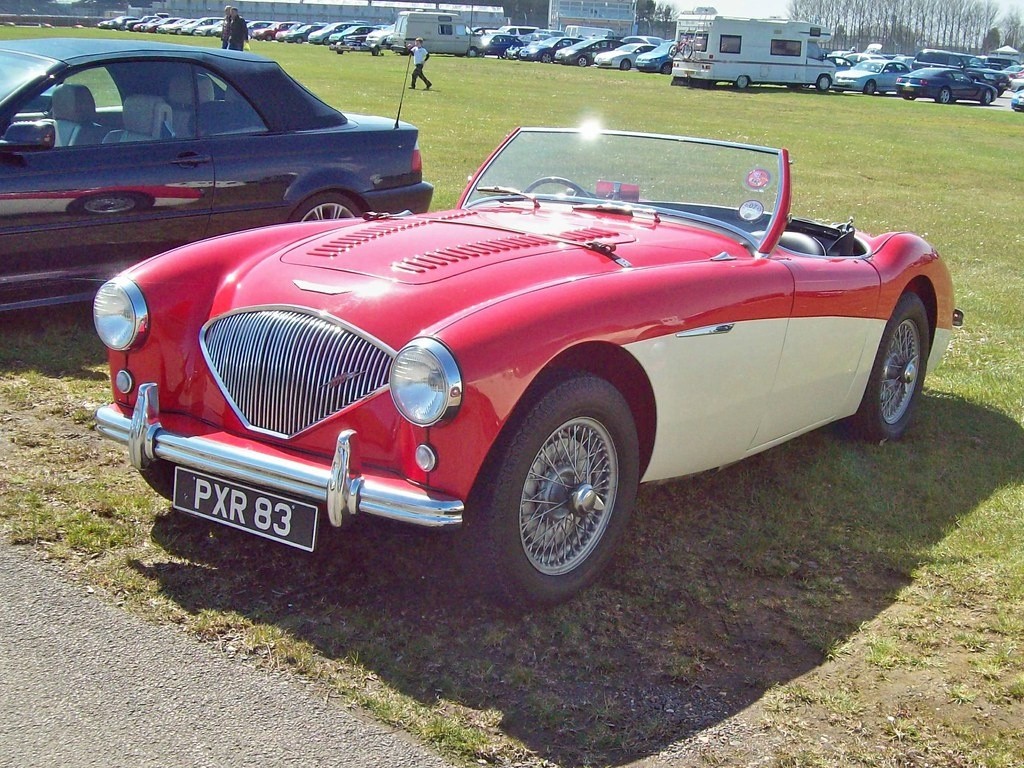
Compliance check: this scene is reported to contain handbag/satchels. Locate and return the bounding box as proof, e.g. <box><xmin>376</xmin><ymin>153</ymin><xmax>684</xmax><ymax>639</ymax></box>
<box><xmin>243</xmin><ymin>43</ymin><xmax>250</xmax><ymax>51</ymax></box>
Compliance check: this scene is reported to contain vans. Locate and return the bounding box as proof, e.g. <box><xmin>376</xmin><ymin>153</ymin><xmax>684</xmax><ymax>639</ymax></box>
<box><xmin>565</xmin><ymin>24</ymin><xmax>617</xmax><ymax>40</ymax></box>
<box><xmin>970</xmin><ymin>55</ymin><xmax>1022</xmax><ymax>71</ymax></box>
<box><xmin>392</xmin><ymin>8</ymin><xmax>484</xmax><ymax>58</ymax></box>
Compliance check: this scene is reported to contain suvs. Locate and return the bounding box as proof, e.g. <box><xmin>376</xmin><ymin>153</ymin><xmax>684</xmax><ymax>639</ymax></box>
<box><xmin>911</xmin><ymin>48</ymin><xmax>1011</xmax><ymax>98</ymax></box>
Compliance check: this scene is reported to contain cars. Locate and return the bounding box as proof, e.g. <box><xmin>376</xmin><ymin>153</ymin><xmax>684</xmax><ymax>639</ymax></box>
<box><xmin>554</xmin><ymin>37</ymin><xmax>629</xmax><ymax>67</ymax></box>
<box><xmin>825</xmin><ymin>42</ymin><xmax>916</xmax><ymax>71</ymax></box>
<box><xmin>1011</xmin><ymin>87</ymin><xmax>1024</xmax><ymax>112</ymax></box>
<box><xmin>834</xmin><ymin>59</ymin><xmax>913</xmax><ymax>96</ymax></box>
<box><xmin>594</xmin><ymin>42</ymin><xmax>658</xmax><ymax>71</ymax></box>
<box><xmin>519</xmin><ymin>29</ymin><xmax>564</xmax><ymax>48</ymax></box>
<box><xmin>97</xmin><ymin>12</ymin><xmax>397</xmax><ymax>53</ymax></box>
<box><xmin>480</xmin><ymin>25</ymin><xmax>540</xmax><ymax>60</ymax></box>
<box><xmin>0</xmin><ymin>37</ymin><xmax>434</xmax><ymax>313</ymax></box>
<box><xmin>635</xmin><ymin>41</ymin><xmax>676</xmax><ymax>76</ymax></box>
<box><xmin>519</xmin><ymin>35</ymin><xmax>587</xmax><ymax>63</ymax></box>
<box><xmin>999</xmin><ymin>64</ymin><xmax>1024</xmax><ymax>93</ymax></box>
<box><xmin>621</xmin><ymin>35</ymin><xmax>667</xmax><ymax>47</ymax></box>
<box><xmin>895</xmin><ymin>66</ymin><xmax>999</xmax><ymax>107</ymax></box>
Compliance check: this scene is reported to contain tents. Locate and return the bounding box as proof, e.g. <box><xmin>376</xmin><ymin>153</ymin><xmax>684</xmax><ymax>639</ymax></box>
<box><xmin>991</xmin><ymin>45</ymin><xmax>1019</xmax><ymax>57</ymax></box>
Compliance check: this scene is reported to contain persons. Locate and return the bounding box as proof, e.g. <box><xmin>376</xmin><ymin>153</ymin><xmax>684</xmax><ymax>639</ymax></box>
<box><xmin>221</xmin><ymin>6</ymin><xmax>249</xmax><ymax>51</ymax></box>
<box><xmin>408</xmin><ymin>37</ymin><xmax>432</xmax><ymax>90</ymax></box>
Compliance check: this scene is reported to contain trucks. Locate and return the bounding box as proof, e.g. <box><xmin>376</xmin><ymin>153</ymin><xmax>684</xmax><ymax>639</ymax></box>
<box><xmin>671</xmin><ymin>6</ymin><xmax>837</xmax><ymax>93</ymax></box>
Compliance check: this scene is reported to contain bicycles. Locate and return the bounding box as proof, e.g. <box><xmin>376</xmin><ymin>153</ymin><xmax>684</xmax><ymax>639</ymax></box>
<box><xmin>667</xmin><ymin>33</ymin><xmax>694</xmax><ymax>60</ymax></box>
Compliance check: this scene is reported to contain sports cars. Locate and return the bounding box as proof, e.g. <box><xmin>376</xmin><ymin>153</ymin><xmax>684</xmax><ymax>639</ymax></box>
<box><xmin>93</xmin><ymin>127</ymin><xmax>966</xmax><ymax>612</ymax></box>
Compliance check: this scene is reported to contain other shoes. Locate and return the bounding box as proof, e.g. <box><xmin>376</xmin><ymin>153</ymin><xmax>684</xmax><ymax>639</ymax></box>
<box><xmin>409</xmin><ymin>86</ymin><xmax>415</xmax><ymax>89</ymax></box>
<box><xmin>424</xmin><ymin>83</ymin><xmax>431</xmax><ymax>90</ymax></box>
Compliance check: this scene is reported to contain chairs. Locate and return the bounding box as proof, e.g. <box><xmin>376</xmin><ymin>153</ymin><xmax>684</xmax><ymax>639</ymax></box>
<box><xmin>750</xmin><ymin>227</ymin><xmax>827</xmax><ymax>257</ymax></box>
<box><xmin>49</xmin><ymin>73</ymin><xmax>263</xmax><ymax>146</ymax></box>
